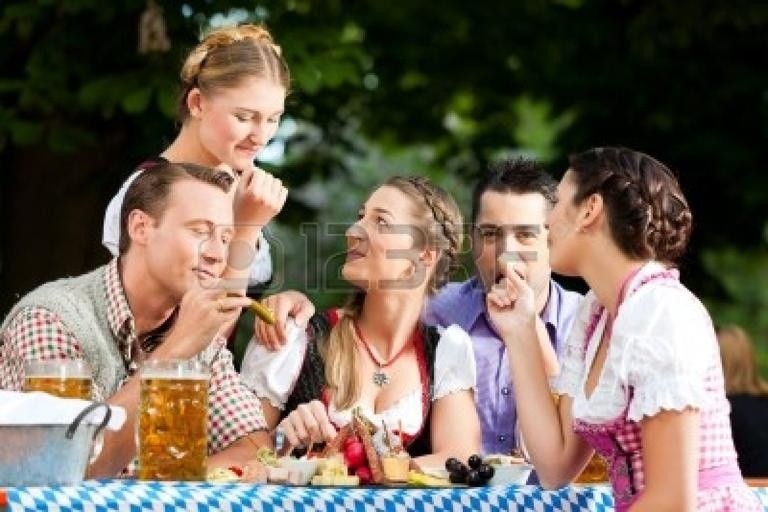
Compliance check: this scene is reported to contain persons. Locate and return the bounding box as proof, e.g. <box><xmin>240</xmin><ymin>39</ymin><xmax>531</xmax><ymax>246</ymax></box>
<box><xmin>102</xmin><ymin>21</ymin><xmax>301</xmax><ymax>374</ymax></box>
<box><xmin>712</xmin><ymin>322</ymin><xmax>768</xmax><ymax>478</ymax></box>
<box><xmin>485</xmin><ymin>146</ymin><xmax>768</xmax><ymax>512</ymax></box>
<box><xmin>0</xmin><ymin>161</ymin><xmax>275</xmax><ymax>488</ymax></box>
<box><xmin>238</xmin><ymin>172</ymin><xmax>484</xmax><ymax>475</ymax></box>
<box><xmin>251</xmin><ymin>153</ymin><xmax>587</xmax><ymax>467</ymax></box>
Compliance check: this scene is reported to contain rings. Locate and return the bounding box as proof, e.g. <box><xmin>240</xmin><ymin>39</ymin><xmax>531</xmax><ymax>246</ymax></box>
<box><xmin>216</xmin><ymin>298</ymin><xmax>227</xmax><ymax>312</ymax></box>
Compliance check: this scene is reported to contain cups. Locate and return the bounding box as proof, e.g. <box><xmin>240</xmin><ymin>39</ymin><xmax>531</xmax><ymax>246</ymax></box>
<box><xmin>136</xmin><ymin>360</ymin><xmax>212</xmax><ymax>482</ymax></box>
<box><xmin>22</xmin><ymin>357</ymin><xmax>93</xmax><ymax>401</ymax></box>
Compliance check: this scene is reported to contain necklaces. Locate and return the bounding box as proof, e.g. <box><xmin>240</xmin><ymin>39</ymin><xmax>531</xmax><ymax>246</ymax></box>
<box><xmin>600</xmin><ymin>261</ymin><xmax>649</xmax><ymax>361</ymax></box>
<box><xmin>348</xmin><ymin>308</ymin><xmax>419</xmax><ymax>391</ymax></box>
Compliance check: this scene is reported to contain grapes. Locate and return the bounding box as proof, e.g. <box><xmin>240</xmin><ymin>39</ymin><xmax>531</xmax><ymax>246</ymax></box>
<box><xmin>445</xmin><ymin>454</ymin><xmax>495</xmax><ymax>488</ymax></box>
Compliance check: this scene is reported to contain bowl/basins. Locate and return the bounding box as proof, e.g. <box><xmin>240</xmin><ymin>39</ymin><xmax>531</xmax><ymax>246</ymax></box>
<box><xmin>1</xmin><ymin>402</ymin><xmax>113</xmax><ymax>485</ymax></box>
<box><xmin>279</xmin><ymin>460</ymin><xmax>317</xmax><ymax>485</ymax></box>
<box><xmin>487</xmin><ymin>464</ymin><xmax>536</xmax><ymax>487</ymax></box>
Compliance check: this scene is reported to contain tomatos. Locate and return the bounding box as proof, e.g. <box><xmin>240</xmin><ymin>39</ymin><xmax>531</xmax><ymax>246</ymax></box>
<box><xmin>342</xmin><ymin>435</ymin><xmax>371</xmax><ymax>483</ymax></box>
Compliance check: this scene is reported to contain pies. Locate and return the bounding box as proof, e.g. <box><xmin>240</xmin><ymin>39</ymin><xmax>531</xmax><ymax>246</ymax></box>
<box><xmin>225</xmin><ymin>293</ymin><xmax>275</xmax><ymax>325</ymax></box>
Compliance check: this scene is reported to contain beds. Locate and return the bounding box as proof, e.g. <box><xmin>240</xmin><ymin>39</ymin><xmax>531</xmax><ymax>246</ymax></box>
<box><xmin>0</xmin><ymin>465</ymin><xmax>618</xmax><ymax>512</ymax></box>
